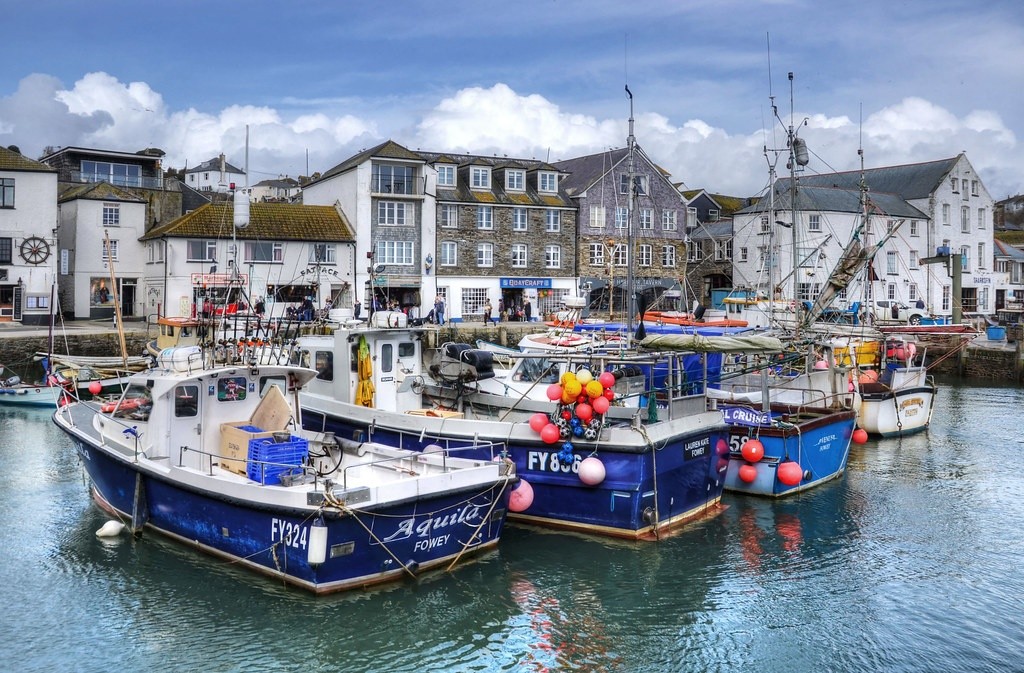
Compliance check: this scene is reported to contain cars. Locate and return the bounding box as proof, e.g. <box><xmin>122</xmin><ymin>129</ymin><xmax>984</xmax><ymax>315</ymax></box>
<box><xmin>862</xmin><ymin>300</ymin><xmax>930</xmax><ymax>324</ymax></box>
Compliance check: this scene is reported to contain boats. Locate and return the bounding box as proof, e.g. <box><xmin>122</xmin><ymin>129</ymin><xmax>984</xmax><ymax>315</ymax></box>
<box><xmin>0</xmin><ymin>231</ymin><xmax>158</xmax><ymax>408</ymax></box>
<box><xmin>49</xmin><ymin>65</ymin><xmax>968</xmax><ymax>594</ymax></box>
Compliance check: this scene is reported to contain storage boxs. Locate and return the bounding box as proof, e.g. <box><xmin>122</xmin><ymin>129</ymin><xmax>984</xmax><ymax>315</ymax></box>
<box><xmin>409</xmin><ymin>409</ymin><xmax>465</xmax><ymax>419</ymax></box>
<box><xmin>987</xmin><ymin>327</ymin><xmax>1005</xmax><ymax>340</ymax></box>
<box><xmin>219</xmin><ymin>383</ymin><xmax>309</xmax><ymax>486</ymax></box>
<box><xmin>615</xmin><ymin>375</ymin><xmax>645</xmax><ymax>394</ymax></box>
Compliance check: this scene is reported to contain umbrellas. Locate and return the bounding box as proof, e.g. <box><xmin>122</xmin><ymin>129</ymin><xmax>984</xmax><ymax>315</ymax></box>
<box><xmin>355</xmin><ymin>336</ymin><xmax>376</xmax><ymax>406</ymax></box>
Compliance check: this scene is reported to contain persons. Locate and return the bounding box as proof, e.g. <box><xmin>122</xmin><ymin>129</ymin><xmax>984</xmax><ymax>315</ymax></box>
<box><xmin>525</xmin><ymin>300</ymin><xmax>531</xmax><ymax>322</ymax></box>
<box><xmin>498</xmin><ymin>299</ymin><xmax>504</xmax><ymax>322</ymax></box>
<box><xmin>354</xmin><ymin>301</ymin><xmax>361</xmax><ymax>319</ymax></box>
<box><xmin>287</xmin><ymin>296</ymin><xmax>332</xmax><ymax>321</ymax></box>
<box><xmin>484</xmin><ymin>298</ymin><xmax>496</xmax><ymax>326</ymax></box>
<box><xmin>916</xmin><ymin>297</ymin><xmax>924</xmax><ymax>309</ymax></box>
<box><xmin>236</xmin><ymin>297</ymin><xmax>265</xmax><ymax>315</ymax></box>
<box><xmin>433</xmin><ymin>293</ymin><xmax>445</xmax><ymax>326</ymax></box>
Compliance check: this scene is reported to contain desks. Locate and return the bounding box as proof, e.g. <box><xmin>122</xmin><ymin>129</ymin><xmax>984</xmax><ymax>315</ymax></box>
<box><xmin>824</xmin><ymin>310</ymin><xmax>846</xmax><ymax>322</ymax></box>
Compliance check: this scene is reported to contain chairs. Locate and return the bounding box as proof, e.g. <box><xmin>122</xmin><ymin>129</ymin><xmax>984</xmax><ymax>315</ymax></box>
<box><xmin>802</xmin><ymin>302</ymin><xmax>862</xmax><ymax>324</ymax></box>
